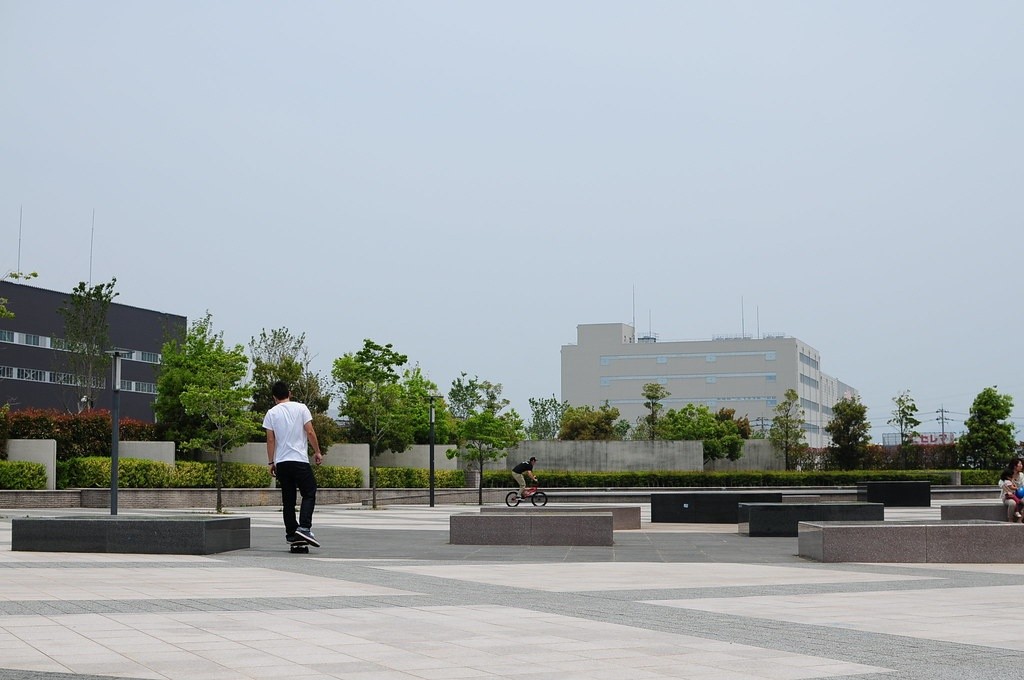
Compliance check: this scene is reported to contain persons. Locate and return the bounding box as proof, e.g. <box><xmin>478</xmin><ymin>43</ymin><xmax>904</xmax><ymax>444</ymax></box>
<box><xmin>512</xmin><ymin>457</ymin><xmax>538</xmax><ymax>501</ymax></box>
<box><xmin>997</xmin><ymin>457</ymin><xmax>1024</xmax><ymax>523</ymax></box>
<box><xmin>262</xmin><ymin>382</ymin><xmax>323</xmax><ymax>547</ymax></box>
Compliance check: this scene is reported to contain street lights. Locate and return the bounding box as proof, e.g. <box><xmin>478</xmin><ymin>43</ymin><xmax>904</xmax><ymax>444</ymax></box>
<box><xmin>104</xmin><ymin>347</ymin><xmax>135</xmax><ymax>516</ymax></box>
<box><xmin>422</xmin><ymin>395</ymin><xmax>444</xmax><ymax>508</ymax></box>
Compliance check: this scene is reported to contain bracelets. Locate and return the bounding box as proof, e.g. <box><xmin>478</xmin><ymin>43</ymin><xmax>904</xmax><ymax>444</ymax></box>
<box><xmin>268</xmin><ymin>462</ymin><xmax>273</xmax><ymax>465</ymax></box>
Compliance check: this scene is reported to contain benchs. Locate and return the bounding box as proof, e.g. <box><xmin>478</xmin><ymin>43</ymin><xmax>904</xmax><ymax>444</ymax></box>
<box><xmin>941</xmin><ymin>504</ymin><xmax>1024</xmax><ymax>523</ymax></box>
<box><xmin>12</xmin><ymin>517</ymin><xmax>251</xmax><ymax>555</ymax></box>
<box><xmin>737</xmin><ymin>502</ymin><xmax>884</xmax><ymax>537</ymax></box>
<box><xmin>651</xmin><ymin>492</ymin><xmax>783</xmax><ymax>525</ymax></box>
<box><xmin>480</xmin><ymin>506</ymin><xmax>641</xmax><ymax>530</ymax></box>
<box><xmin>450</xmin><ymin>511</ymin><xmax>614</xmax><ymax>546</ymax></box>
<box><xmin>798</xmin><ymin>520</ymin><xmax>1024</xmax><ymax>563</ymax></box>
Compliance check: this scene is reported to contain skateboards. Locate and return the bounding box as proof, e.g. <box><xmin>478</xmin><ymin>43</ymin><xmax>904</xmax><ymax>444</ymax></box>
<box><xmin>286</xmin><ymin>541</ymin><xmax>309</xmax><ymax>554</ymax></box>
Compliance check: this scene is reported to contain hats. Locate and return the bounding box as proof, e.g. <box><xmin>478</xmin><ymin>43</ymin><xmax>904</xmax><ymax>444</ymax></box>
<box><xmin>530</xmin><ymin>457</ymin><xmax>538</xmax><ymax>461</ymax></box>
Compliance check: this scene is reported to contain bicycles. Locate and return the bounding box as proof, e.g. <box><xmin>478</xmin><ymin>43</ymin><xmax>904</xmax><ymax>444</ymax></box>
<box><xmin>505</xmin><ymin>475</ymin><xmax>548</xmax><ymax>507</ymax></box>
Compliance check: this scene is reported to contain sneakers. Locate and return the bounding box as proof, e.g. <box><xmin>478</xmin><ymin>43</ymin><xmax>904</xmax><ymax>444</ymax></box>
<box><xmin>295</xmin><ymin>529</ymin><xmax>320</xmax><ymax>548</ymax></box>
<box><xmin>285</xmin><ymin>536</ymin><xmax>307</xmax><ymax>544</ymax></box>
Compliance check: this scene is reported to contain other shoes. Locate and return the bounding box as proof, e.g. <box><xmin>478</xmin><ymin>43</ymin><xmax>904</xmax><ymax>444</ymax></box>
<box><xmin>1015</xmin><ymin>511</ymin><xmax>1022</xmax><ymax>517</ymax></box>
<box><xmin>516</xmin><ymin>497</ymin><xmax>526</xmax><ymax>501</ymax></box>
